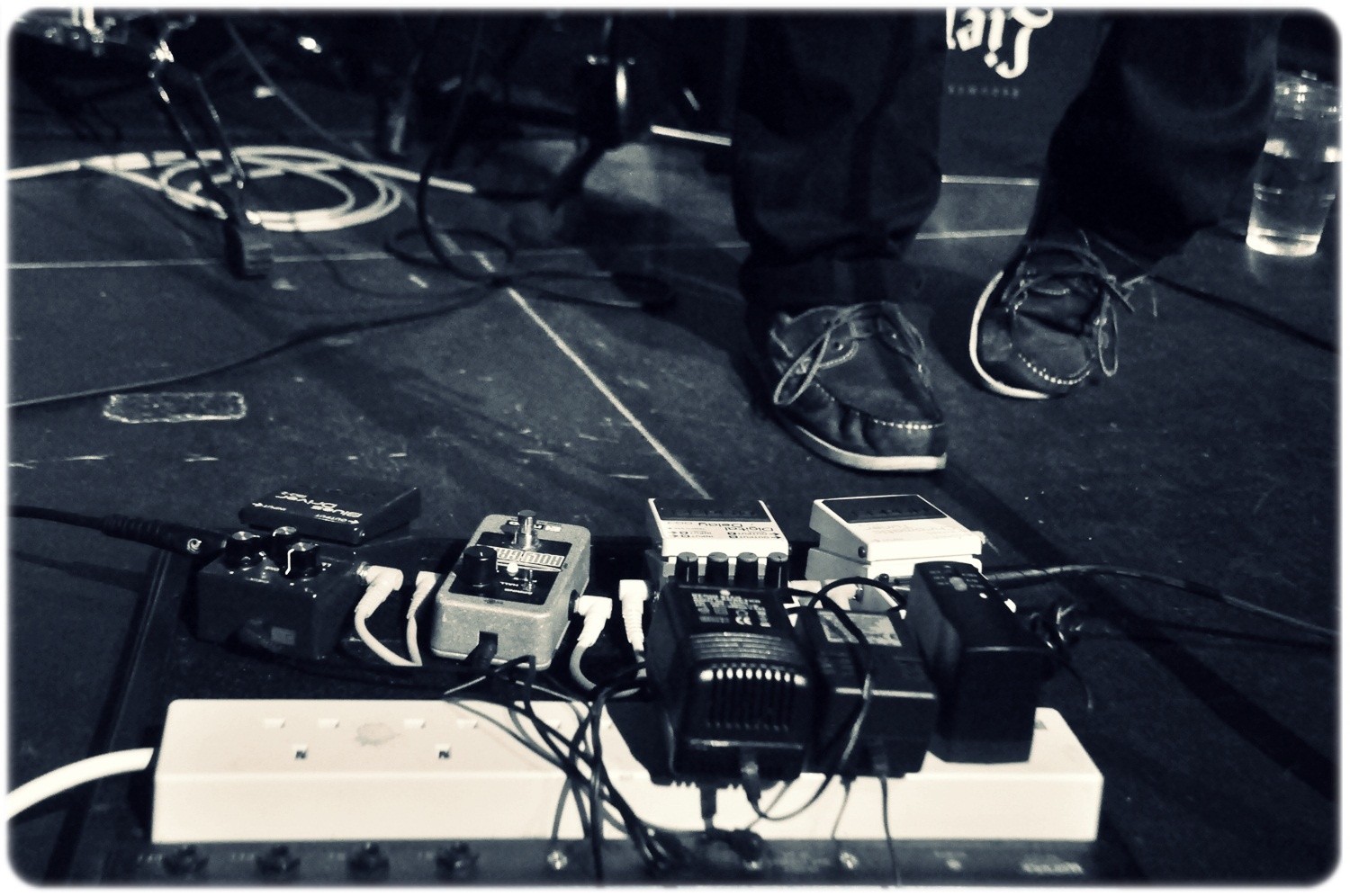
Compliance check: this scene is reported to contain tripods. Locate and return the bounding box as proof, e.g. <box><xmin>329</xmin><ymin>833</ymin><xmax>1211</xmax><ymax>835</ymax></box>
<box><xmin>462</xmin><ymin>10</ymin><xmax>737</xmax><ymax>244</ymax></box>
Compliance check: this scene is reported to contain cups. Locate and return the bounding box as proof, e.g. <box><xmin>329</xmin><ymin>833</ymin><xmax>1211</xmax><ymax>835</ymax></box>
<box><xmin>1243</xmin><ymin>69</ymin><xmax>1341</xmax><ymax>258</ymax></box>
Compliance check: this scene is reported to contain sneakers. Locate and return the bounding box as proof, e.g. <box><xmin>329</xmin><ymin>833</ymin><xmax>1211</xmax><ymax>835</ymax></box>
<box><xmin>754</xmin><ymin>300</ymin><xmax>948</xmax><ymax>473</ymax></box>
<box><xmin>968</xmin><ymin>151</ymin><xmax>1159</xmax><ymax>401</ymax></box>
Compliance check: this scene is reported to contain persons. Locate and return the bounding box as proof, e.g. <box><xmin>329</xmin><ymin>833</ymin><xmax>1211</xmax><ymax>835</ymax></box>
<box><xmin>718</xmin><ymin>7</ymin><xmax>1285</xmax><ymax>472</ymax></box>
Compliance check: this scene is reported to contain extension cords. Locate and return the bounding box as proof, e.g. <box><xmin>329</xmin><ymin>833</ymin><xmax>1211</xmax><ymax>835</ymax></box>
<box><xmin>154</xmin><ymin>698</ymin><xmax>1103</xmax><ymax>844</ymax></box>
